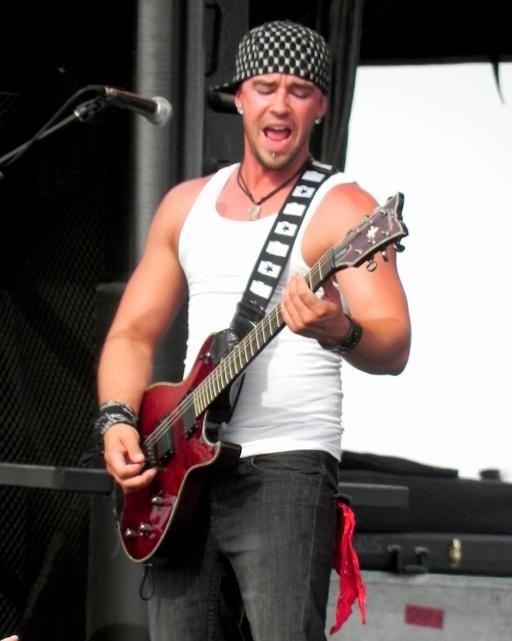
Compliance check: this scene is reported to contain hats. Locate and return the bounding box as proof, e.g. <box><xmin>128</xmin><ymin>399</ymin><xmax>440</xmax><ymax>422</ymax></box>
<box><xmin>207</xmin><ymin>20</ymin><xmax>333</xmax><ymax>95</ymax></box>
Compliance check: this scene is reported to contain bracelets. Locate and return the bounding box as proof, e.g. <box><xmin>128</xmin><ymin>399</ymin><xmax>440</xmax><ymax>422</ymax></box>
<box><xmin>95</xmin><ymin>400</ymin><xmax>139</xmax><ymax>436</ymax></box>
<box><xmin>318</xmin><ymin>314</ymin><xmax>362</xmax><ymax>355</ymax></box>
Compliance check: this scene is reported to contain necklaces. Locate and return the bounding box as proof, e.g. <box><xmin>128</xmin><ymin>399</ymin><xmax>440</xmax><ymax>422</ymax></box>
<box><xmin>236</xmin><ymin>153</ymin><xmax>311</xmax><ymax>222</ymax></box>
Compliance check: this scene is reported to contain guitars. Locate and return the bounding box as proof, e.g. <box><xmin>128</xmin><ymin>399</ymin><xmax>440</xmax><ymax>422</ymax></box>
<box><xmin>112</xmin><ymin>193</ymin><xmax>407</xmax><ymax>562</ymax></box>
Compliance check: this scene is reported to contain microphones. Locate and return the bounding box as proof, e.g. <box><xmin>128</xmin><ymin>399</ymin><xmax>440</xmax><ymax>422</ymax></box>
<box><xmin>90</xmin><ymin>85</ymin><xmax>173</xmax><ymax>128</ymax></box>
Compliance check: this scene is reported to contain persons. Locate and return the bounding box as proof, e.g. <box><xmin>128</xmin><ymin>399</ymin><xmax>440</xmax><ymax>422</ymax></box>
<box><xmin>96</xmin><ymin>21</ymin><xmax>410</xmax><ymax>641</ymax></box>
